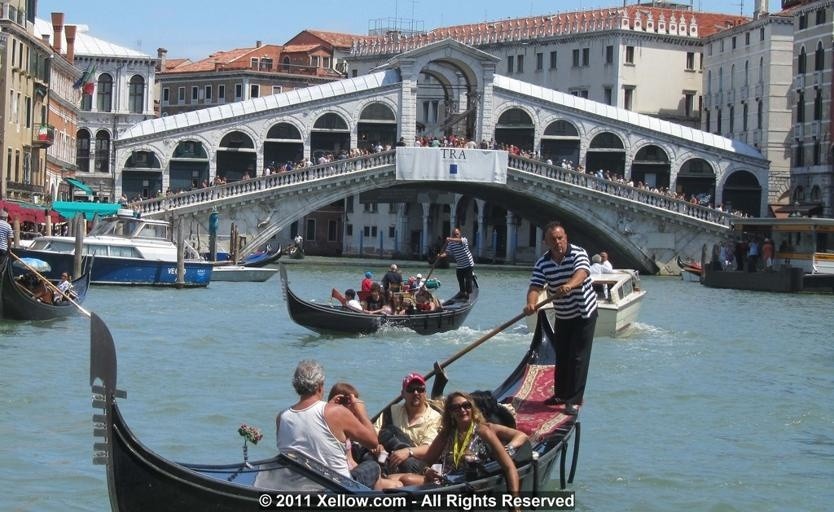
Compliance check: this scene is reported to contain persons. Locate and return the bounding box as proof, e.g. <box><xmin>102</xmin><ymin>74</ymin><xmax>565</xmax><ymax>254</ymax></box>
<box><xmin>275</xmin><ymin>357</ymin><xmax>530</xmax><ymax>512</ymax></box>
<box><xmin>0</xmin><ymin>130</ymin><xmax>794</xmax><ymax>318</ymax></box>
<box><xmin>524</xmin><ymin>222</ymin><xmax>597</xmax><ymax>416</ymax></box>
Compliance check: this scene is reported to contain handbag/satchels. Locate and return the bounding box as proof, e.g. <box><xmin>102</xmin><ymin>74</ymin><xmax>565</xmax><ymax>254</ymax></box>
<box><xmin>378</xmin><ymin>425</ymin><xmax>416</xmax><ymax>453</ymax></box>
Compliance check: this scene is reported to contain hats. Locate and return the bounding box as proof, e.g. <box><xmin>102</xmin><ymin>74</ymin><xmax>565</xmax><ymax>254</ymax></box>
<box><xmin>403</xmin><ymin>372</ymin><xmax>426</xmax><ymax>389</ymax></box>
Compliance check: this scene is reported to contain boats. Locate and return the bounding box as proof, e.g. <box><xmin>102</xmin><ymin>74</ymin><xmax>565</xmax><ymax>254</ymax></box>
<box><xmin>10</xmin><ymin>217</ymin><xmax>214</xmax><ymax>289</ymax></box>
<box><xmin>89</xmin><ymin>310</ymin><xmax>584</xmax><ymax>511</ymax></box>
<box><xmin>198</xmin><ymin>241</ymin><xmax>282</xmax><ymax>282</ymax></box>
<box><xmin>525</xmin><ymin>268</ymin><xmax>648</xmax><ymax>339</ymax></box>
<box><xmin>676</xmin><ymin>217</ymin><xmax>834</xmax><ymax>291</ymax></box>
<box><xmin>701</xmin><ymin>264</ymin><xmax>804</xmax><ymax>294</ymax></box>
<box><xmin>288</xmin><ymin>242</ymin><xmax>307</xmax><ymax>259</ymax></box>
<box><xmin>279</xmin><ymin>264</ymin><xmax>481</xmax><ymax>336</ymax></box>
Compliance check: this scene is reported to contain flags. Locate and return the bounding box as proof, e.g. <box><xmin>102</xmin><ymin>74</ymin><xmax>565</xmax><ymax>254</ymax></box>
<box><xmin>69</xmin><ymin>61</ymin><xmax>98</xmax><ymax>97</ymax></box>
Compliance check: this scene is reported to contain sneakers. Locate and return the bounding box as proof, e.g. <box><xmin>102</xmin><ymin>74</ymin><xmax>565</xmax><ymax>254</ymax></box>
<box><xmin>564</xmin><ymin>404</ymin><xmax>580</xmax><ymax>415</ymax></box>
<box><xmin>544</xmin><ymin>395</ymin><xmax>566</xmax><ymax>405</ymax></box>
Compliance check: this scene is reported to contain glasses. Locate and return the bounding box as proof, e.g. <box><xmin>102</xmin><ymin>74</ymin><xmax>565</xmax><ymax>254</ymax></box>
<box><xmin>446</xmin><ymin>401</ymin><xmax>473</xmax><ymax>413</ymax></box>
<box><xmin>406</xmin><ymin>386</ymin><xmax>426</xmax><ymax>393</ymax></box>
